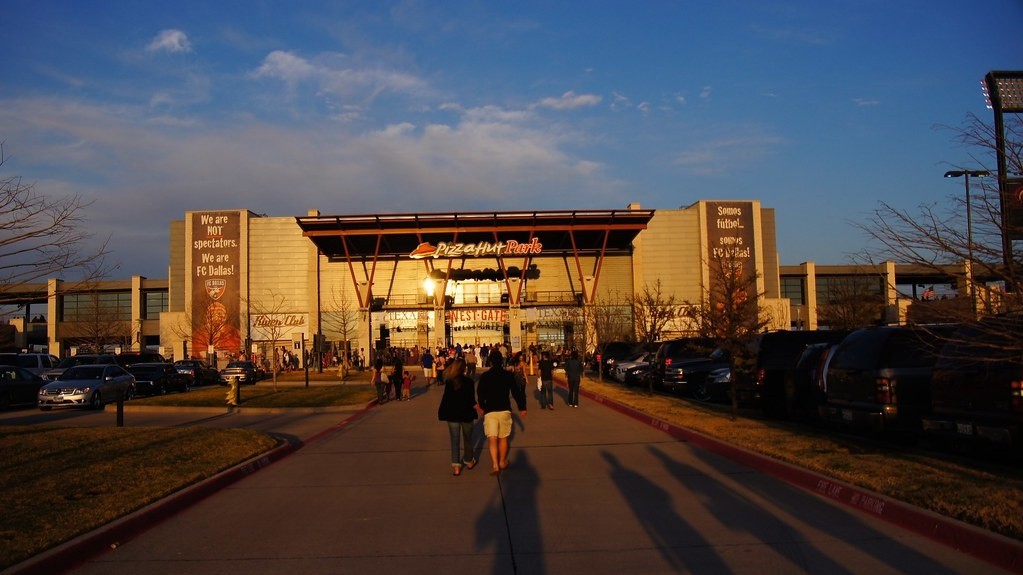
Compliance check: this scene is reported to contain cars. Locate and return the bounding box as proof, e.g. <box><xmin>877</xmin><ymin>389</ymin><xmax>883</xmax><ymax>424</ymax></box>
<box><xmin>591</xmin><ymin>323</ymin><xmax>1022</xmax><ymax>462</ymax></box>
<box><xmin>1</xmin><ymin>359</ymin><xmax>266</xmax><ymax>419</ymax></box>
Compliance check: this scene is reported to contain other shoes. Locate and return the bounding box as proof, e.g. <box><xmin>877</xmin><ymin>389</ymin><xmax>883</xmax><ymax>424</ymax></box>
<box><xmin>407</xmin><ymin>399</ymin><xmax>411</xmax><ymax>401</ymax></box>
<box><xmin>568</xmin><ymin>403</ymin><xmax>577</xmax><ymax>407</ymax></box>
<box><xmin>396</xmin><ymin>397</ymin><xmax>401</xmax><ymax>401</ymax></box>
<box><xmin>402</xmin><ymin>398</ymin><xmax>406</xmax><ymax>400</ymax></box>
<box><xmin>543</xmin><ymin>403</ymin><xmax>554</xmax><ymax>410</ymax></box>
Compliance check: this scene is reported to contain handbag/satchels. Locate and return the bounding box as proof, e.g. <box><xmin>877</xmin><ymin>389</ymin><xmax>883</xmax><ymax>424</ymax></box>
<box><xmin>437</xmin><ymin>361</ymin><xmax>442</xmax><ymax>367</ymax></box>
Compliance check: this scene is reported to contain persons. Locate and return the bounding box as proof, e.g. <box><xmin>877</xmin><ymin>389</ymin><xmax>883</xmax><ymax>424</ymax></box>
<box><xmin>167</xmin><ymin>332</ymin><xmax>597</xmax><ymax>413</ymax></box>
<box><xmin>437</xmin><ymin>358</ymin><xmax>483</xmax><ymax>475</ymax></box>
<box><xmin>476</xmin><ymin>350</ymin><xmax>527</xmax><ymax>475</ymax></box>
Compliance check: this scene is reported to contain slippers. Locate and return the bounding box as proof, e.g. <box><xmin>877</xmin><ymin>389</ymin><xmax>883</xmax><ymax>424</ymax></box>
<box><xmin>490</xmin><ymin>460</ymin><xmax>509</xmax><ymax>475</ymax></box>
<box><xmin>454</xmin><ymin>459</ymin><xmax>477</xmax><ymax>476</ymax></box>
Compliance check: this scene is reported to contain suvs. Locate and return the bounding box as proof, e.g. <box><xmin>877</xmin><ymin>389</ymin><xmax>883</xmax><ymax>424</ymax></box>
<box><xmin>39</xmin><ymin>352</ymin><xmax>168</xmax><ymax>381</ymax></box>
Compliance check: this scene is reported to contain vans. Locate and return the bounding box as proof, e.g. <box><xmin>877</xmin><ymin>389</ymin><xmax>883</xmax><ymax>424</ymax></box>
<box><xmin>2</xmin><ymin>352</ymin><xmax>60</xmax><ymax>376</ymax></box>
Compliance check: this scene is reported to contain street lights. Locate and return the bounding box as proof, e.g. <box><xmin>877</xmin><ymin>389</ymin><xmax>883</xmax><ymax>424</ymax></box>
<box><xmin>943</xmin><ymin>167</ymin><xmax>991</xmax><ymax>320</ymax></box>
